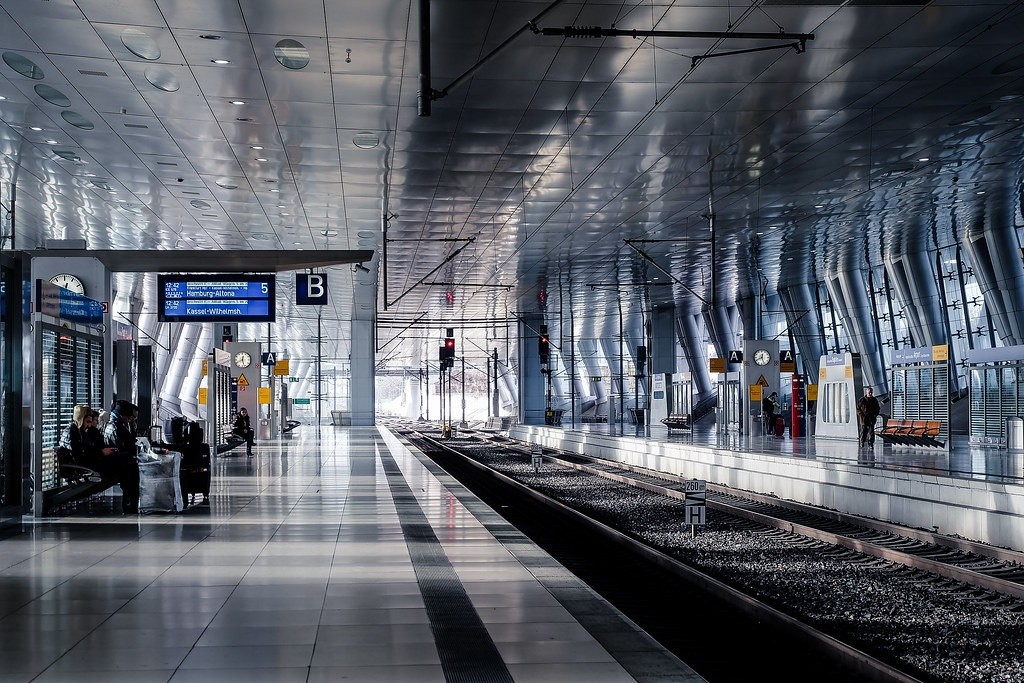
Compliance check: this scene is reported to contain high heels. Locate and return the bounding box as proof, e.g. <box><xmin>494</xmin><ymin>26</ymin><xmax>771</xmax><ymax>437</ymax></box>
<box><xmin>247</xmin><ymin>452</ymin><xmax>254</xmax><ymax>457</ymax></box>
<box><xmin>251</xmin><ymin>440</ymin><xmax>257</xmax><ymax>446</ymax></box>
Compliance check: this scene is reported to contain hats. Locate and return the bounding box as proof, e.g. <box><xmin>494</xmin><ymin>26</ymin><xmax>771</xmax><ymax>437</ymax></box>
<box><xmin>771</xmin><ymin>392</ymin><xmax>778</xmax><ymax>397</ymax></box>
<box><xmin>72</xmin><ymin>405</ymin><xmax>93</xmax><ymax>429</ymax></box>
<box><xmin>116</xmin><ymin>400</ymin><xmax>137</xmax><ymax>418</ymax></box>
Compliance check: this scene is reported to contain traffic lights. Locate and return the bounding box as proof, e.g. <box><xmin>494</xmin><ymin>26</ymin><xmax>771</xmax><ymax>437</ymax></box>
<box><xmin>538</xmin><ymin>334</ymin><xmax>549</xmax><ymax>354</ymax></box>
<box><xmin>445</xmin><ymin>337</ymin><xmax>455</xmax><ymax>358</ymax></box>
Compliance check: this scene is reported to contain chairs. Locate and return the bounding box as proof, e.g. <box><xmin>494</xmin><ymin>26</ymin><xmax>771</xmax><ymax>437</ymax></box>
<box><xmin>660</xmin><ymin>413</ymin><xmax>691</xmax><ymax>430</ymax></box>
<box><xmin>283</xmin><ymin>416</ymin><xmax>301</xmax><ymax>433</ymax></box>
<box><xmin>217</xmin><ymin>423</ymin><xmax>246</xmax><ymax>455</ymax></box>
<box><xmin>874</xmin><ymin>419</ymin><xmax>945</xmax><ymax>448</ymax></box>
<box><xmin>45</xmin><ymin>446</ymin><xmax>117</xmax><ymax>512</ymax></box>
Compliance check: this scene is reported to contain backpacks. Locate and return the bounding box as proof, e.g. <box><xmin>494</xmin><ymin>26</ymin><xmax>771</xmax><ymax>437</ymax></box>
<box><xmin>763</xmin><ymin>397</ymin><xmax>767</xmax><ymax>406</ymax></box>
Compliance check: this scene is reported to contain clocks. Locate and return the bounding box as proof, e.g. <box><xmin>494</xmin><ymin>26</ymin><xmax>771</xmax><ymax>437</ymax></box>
<box><xmin>234</xmin><ymin>351</ymin><xmax>251</xmax><ymax>369</ymax></box>
<box><xmin>49</xmin><ymin>273</ymin><xmax>85</xmax><ymax>296</ymax></box>
<box><xmin>753</xmin><ymin>349</ymin><xmax>771</xmax><ymax>366</ymax></box>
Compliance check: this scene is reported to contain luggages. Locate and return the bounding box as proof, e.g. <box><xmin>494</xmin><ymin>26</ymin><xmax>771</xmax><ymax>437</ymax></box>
<box><xmin>135</xmin><ymin>425</ymin><xmax>184</xmax><ymax>514</ymax></box>
<box><xmin>181</xmin><ymin>443</ymin><xmax>211</xmax><ymax>497</ymax></box>
<box><xmin>773</xmin><ymin>407</ymin><xmax>785</xmax><ymax>436</ymax></box>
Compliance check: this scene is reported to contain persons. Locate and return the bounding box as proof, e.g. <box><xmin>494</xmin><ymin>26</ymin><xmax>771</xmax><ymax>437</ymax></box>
<box><xmin>856</xmin><ymin>388</ymin><xmax>880</xmax><ymax>449</ymax></box>
<box><xmin>58</xmin><ymin>400</ymin><xmax>141</xmax><ymax>511</ymax></box>
<box><xmin>764</xmin><ymin>392</ymin><xmax>781</xmax><ymax>437</ymax></box>
<box><xmin>231</xmin><ymin>407</ymin><xmax>257</xmax><ymax>457</ymax></box>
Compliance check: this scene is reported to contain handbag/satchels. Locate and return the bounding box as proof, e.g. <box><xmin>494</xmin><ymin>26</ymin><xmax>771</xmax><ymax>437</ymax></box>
<box><xmin>225</xmin><ymin>435</ymin><xmax>243</xmax><ymax>444</ymax></box>
<box><xmin>183</xmin><ymin>421</ymin><xmax>204</xmax><ymax>442</ymax></box>
<box><xmin>171</xmin><ymin>416</ymin><xmax>188</xmax><ymax>442</ymax></box>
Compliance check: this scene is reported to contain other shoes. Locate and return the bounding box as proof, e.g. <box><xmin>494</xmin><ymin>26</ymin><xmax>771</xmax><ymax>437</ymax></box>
<box><xmin>767</xmin><ymin>433</ymin><xmax>773</xmax><ymax>436</ymax></box>
<box><xmin>869</xmin><ymin>443</ymin><xmax>874</xmax><ymax>449</ymax></box>
<box><xmin>860</xmin><ymin>441</ymin><xmax>864</xmax><ymax>447</ymax></box>
